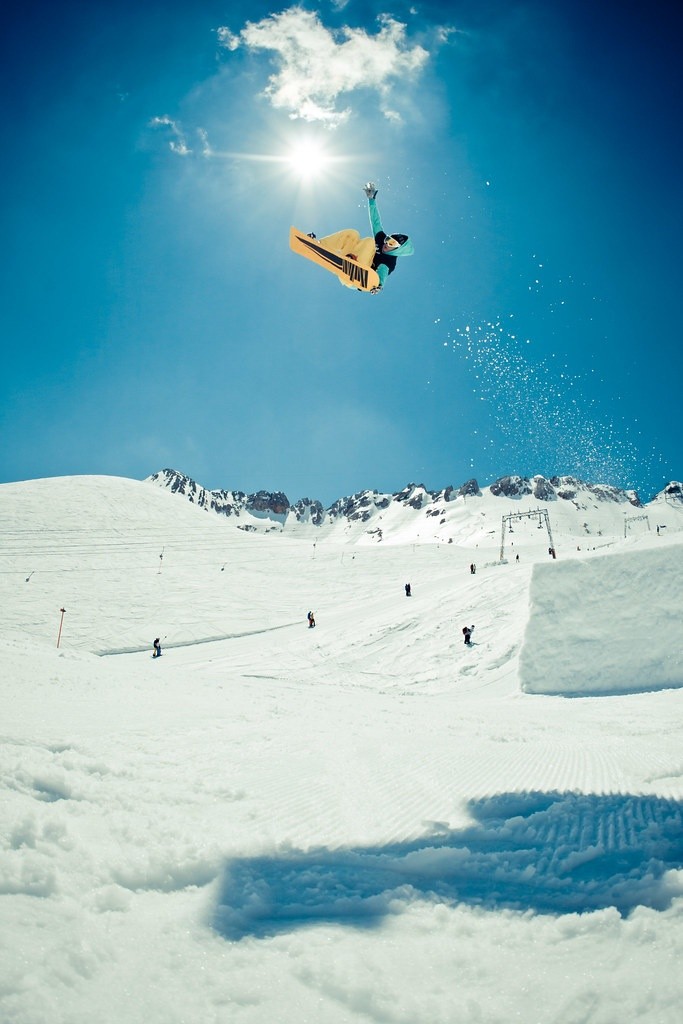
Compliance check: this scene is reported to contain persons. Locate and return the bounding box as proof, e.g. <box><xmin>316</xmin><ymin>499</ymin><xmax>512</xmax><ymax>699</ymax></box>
<box><xmin>307</xmin><ymin>611</ymin><xmax>315</xmax><ymax>628</ymax></box>
<box><xmin>405</xmin><ymin>583</ymin><xmax>411</xmax><ymax>596</ymax></box>
<box><xmin>470</xmin><ymin>563</ymin><xmax>476</xmax><ymax>573</ymax></box>
<box><xmin>307</xmin><ymin>182</ymin><xmax>414</xmax><ymax>295</ymax></box>
<box><xmin>657</xmin><ymin>524</ymin><xmax>659</xmax><ymax>535</ymax></box>
<box><xmin>515</xmin><ymin>555</ymin><xmax>518</xmax><ymax>562</ymax></box>
<box><xmin>463</xmin><ymin>625</ymin><xmax>475</xmax><ymax>644</ymax></box>
<box><xmin>153</xmin><ymin>638</ymin><xmax>162</xmax><ymax>657</ymax></box>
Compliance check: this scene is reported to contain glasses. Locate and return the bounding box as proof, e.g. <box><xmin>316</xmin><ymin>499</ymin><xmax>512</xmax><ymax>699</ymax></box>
<box><xmin>384</xmin><ymin>235</ymin><xmax>402</xmax><ymax>248</ymax></box>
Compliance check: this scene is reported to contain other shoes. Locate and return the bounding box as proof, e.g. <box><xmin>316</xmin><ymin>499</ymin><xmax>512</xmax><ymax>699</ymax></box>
<box><xmin>307</xmin><ymin>232</ymin><xmax>316</xmax><ymax>240</ymax></box>
<box><xmin>346</xmin><ymin>253</ymin><xmax>357</xmax><ymax>260</ymax></box>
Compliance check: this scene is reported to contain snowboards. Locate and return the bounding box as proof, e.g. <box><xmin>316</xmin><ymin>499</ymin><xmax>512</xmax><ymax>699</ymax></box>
<box><xmin>290</xmin><ymin>226</ymin><xmax>380</xmax><ymax>291</ymax></box>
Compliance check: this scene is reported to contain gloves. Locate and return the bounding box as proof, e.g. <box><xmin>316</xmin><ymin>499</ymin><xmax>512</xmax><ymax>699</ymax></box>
<box><xmin>363</xmin><ymin>182</ymin><xmax>378</xmax><ymax>197</ymax></box>
<box><xmin>370</xmin><ymin>288</ymin><xmax>380</xmax><ymax>295</ymax></box>
